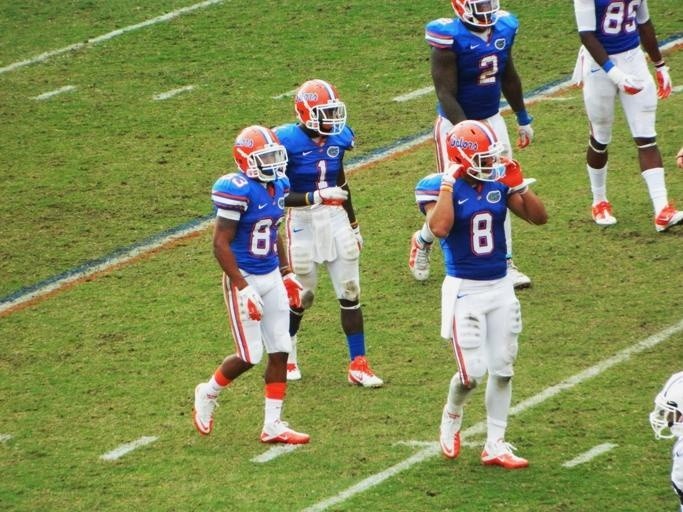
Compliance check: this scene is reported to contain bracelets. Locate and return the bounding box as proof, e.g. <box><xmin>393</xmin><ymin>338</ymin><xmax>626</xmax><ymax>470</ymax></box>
<box><xmin>516</xmin><ymin>110</ymin><xmax>532</xmax><ymax>126</ymax></box>
<box><xmin>351</xmin><ymin>221</ymin><xmax>357</xmax><ymax>228</ymax></box>
<box><xmin>440</xmin><ymin>182</ymin><xmax>454</xmax><ymax>193</ymax></box>
<box><xmin>602</xmin><ymin>60</ymin><xmax>614</xmax><ymax>73</ymax></box>
<box><xmin>305</xmin><ymin>190</ymin><xmax>313</xmax><ymax>206</ymax></box>
<box><xmin>280</xmin><ymin>265</ymin><xmax>291</xmax><ymax>275</ymax></box>
<box><xmin>520</xmin><ymin>185</ymin><xmax>528</xmax><ymax>194</ymax></box>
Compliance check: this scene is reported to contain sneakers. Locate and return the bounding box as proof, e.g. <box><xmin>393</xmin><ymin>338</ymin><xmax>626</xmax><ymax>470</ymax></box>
<box><xmin>505</xmin><ymin>258</ymin><xmax>533</xmax><ymax>288</ymax></box>
<box><xmin>438</xmin><ymin>403</ymin><xmax>465</xmax><ymax>461</ymax></box>
<box><xmin>653</xmin><ymin>206</ymin><xmax>683</xmax><ymax>234</ymax></box>
<box><xmin>407</xmin><ymin>229</ymin><xmax>434</xmax><ymax>282</ymax></box>
<box><xmin>591</xmin><ymin>200</ymin><xmax>618</xmax><ymax>226</ymax></box>
<box><xmin>348</xmin><ymin>355</ymin><xmax>387</xmax><ymax>389</ymax></box>
<box><xmin>260</xmin><ymin>421</ymin><xmax>311</xmax><ymax>443</ymax></box>
<box><xmin>285</xmin><ymin>345</ymin><xmax>302</xmax><ymax>381</ymax></box>
<box><xmin>478</xmin><ymin>440</ymin><xmax>529</xmax><ymax>472</ymax></box>
<box><xmin>191</xmin><ymin>380</ymin><xmax>218</xmax><ymax>436</ymax></box>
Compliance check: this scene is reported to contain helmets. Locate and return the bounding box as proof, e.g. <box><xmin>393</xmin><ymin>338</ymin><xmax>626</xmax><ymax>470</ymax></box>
<box><xmin>649</xmin><ymin>367</ymin><xmax>683</xmax><ymax>442</ymax></box>
<box><xmin>294</xmin><ymin>78</ymin><xmax>347</xmax><ymax>136</ymax></box>
<box><xmin>447</xmin><ymin>119</ymin><xmax>508</xmax><ymax>182</ymax></box>
<box><xmin>233</xmin><ymin>124</ymin><xmax>289</xmax><ymax>182</ymax></box>
<box><xmin>449</xmin><ymin>1</ymin><xmax>503</xmax><ymax>28</ymax></box>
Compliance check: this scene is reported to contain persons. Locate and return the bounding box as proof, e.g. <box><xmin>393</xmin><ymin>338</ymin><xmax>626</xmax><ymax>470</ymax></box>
<box><xmin>649</xmin><ymin>372</ymin><xmax>682</xmax><ymax>511</ymax></box>
<box><xmin>409</xmin><ymin>0</ymin><xmax>536</xmax><ymax>287</ymax></box>
<box><xmin>272</xmin><ymin>80</ymin><xmax>385</xmax><ymax>387</ymax></box>
<box><xmin>415</xmin><ymin>120</ymin><xmax>548</xmax><ymax>469</ymax></box>
<box><xmin>192</xmin><ymin>126</ymin><xmax>310</xmax><ymax>444</ymax></box>
<box><xmin>572</xmin><ymin>1</ymin><xmax>683</xmax><ymax>232</ymax></box>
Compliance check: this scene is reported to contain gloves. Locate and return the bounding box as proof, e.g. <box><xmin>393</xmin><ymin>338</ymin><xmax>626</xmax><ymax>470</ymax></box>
<box><xmin>439</xmin><ymin>164</ymin><xmax>466</xmax><ymax>187</ymax></box>
<box><xmin>353</xmin><ymin>224</ymin><xmax>363</xmax><ymax>253</ymax></box>
<box><xmin>238</xmin><ymin>284</ymin><xmax>266</xmax><ymax>322</ymax></box>
<box><xmin>515</xmin><ymin>121</ymin><xmax>535</xmax><ymax>151</ymax></box>
<box><xmin>653</xmin><ymin>61</ymin><xmax>674</xmax><ymax>100</ymax></box>
<box><xmin>281</xmin><ymin>271</ymin><xmax>306</xmax><ymax>309</ymax></box>
<box><xmin>312</xmin><ymin>186</ymin><xmax>349</xmax><ymax>206</ymax></box>
<box><xmin>493</xmin><ymin>154</ymin><xmax>524</xmax><ymax>191</ymax></box>
<box><xmin>606</xmin><ymin>64</ymin><xmax>646</xmax><ymax>96</ymax></box>
